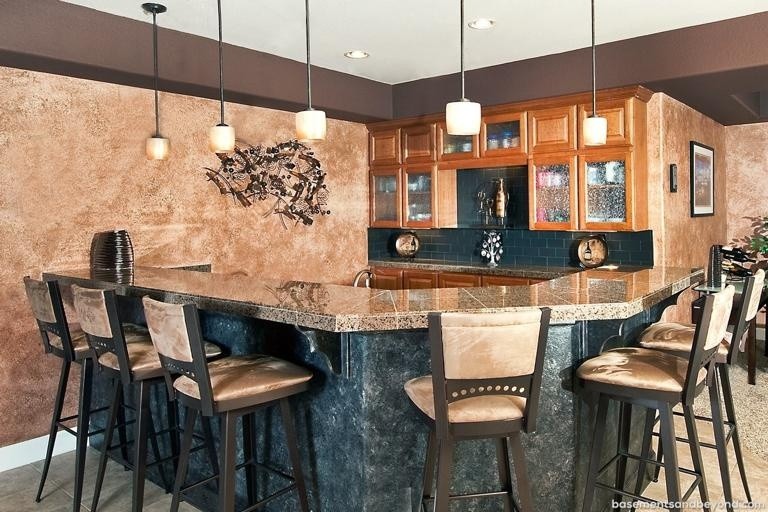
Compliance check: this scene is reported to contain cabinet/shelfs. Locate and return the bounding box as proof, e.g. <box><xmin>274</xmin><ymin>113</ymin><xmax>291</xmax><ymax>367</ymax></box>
<box><xmin>436</xmin><ymin>101</ymin><xmax>527</xmax><ymax>169</ymax></box>
<box><xmin>439</xmin><ymin>273</ymin><xmax>528</xmax><ymax>288</ymax></box>
<box><xmin>371</xmin><ymin>269</ymin><xmax>438</xmax><ymax>290</ymax></box>
<box><xmin>526</xmin><ymin>85</ymin><xmax>653</xmax><ymax>232</ymax></box>
<box><xmin>366</xmin><ymin>113</ymin><xmax>457</xmax><ymax>231</ymax></box>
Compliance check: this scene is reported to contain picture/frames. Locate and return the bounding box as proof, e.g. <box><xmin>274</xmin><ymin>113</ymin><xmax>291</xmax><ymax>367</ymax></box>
<box><xmin>690</xmin><ymin>140</ymin><xmax>715</xmax><ymax>217</ymax></box>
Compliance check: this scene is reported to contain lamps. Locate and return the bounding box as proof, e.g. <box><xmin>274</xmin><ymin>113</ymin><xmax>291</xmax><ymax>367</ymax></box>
<box><xmin>140</xmin><ymin>0</ymin><xmax>328</xmax><ymax>161</ymax></box>
<box><xmin>583</xmin><ymin>1</ymin><xmax>609</xmax><ymax>147</ymax></box>
<box><xmin>445</xmin><ymin>0</ymin><xmax>482</xmax><ymax>136</ymax></box>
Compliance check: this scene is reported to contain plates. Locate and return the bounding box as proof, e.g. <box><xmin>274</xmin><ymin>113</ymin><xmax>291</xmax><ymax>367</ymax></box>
<box><xmin>576</xmin><ymin>235</ymin><xmax>608</xmax><ymax>268</ymax></box>
<box><xmin>393</xmin><ymin>232</ymin><xmax>419</xmax><ymax>257</ymax></box>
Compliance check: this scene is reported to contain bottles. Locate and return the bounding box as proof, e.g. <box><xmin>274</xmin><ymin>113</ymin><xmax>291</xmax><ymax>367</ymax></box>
<box><xmin>441</xmin><ymin>132</ymin><xmax>513</xmax><ymax>154</ymax></box>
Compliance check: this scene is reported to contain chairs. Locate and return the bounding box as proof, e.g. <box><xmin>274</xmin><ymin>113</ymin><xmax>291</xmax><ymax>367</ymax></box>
<box><xmin>404</xmin><ymin>306</ymin><xmax>551</xmax><ymax>510</ymax></box>
